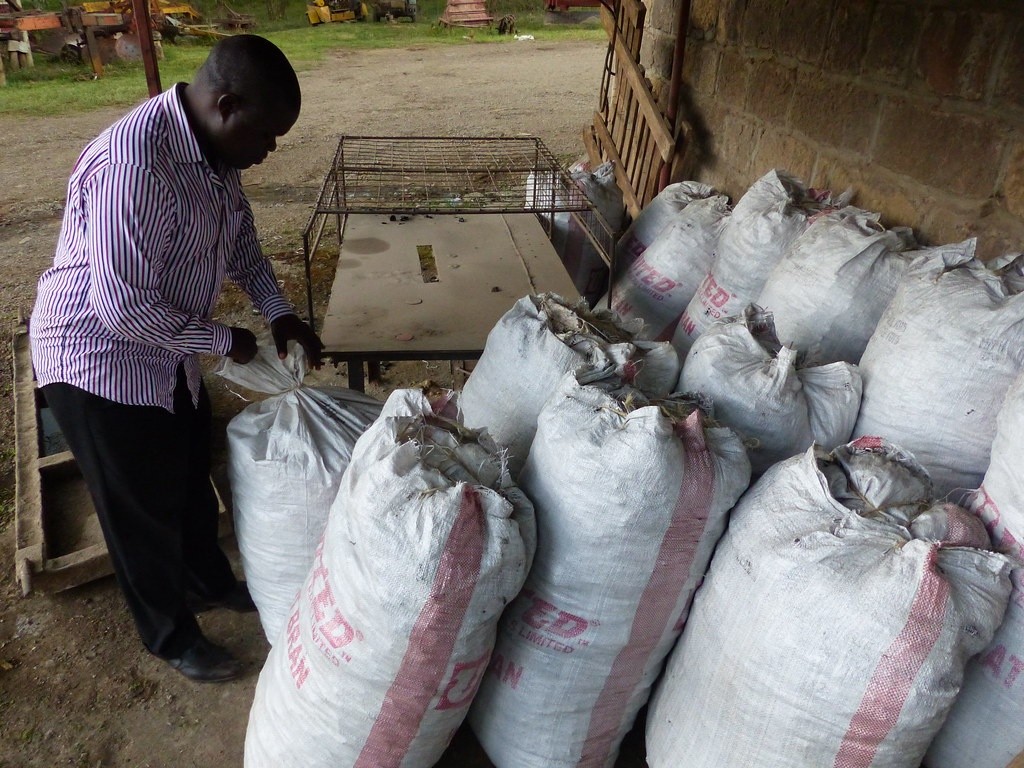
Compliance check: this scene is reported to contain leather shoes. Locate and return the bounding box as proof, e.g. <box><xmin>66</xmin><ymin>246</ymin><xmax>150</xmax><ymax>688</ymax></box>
<box><xmin>166</xmin><ymin>634</ymin><xmax>247</xmax><ymax>683</ymax></box>
<box><xmin>223</xmin><ymin>581</ymin><xmax>258</xmax><ymax>612</ymax></box>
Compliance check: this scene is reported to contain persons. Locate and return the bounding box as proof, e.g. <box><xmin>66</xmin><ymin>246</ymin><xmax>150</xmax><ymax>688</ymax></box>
<box><xmin>30</xmin><ymin>34</ymin><xmax>326</xmax><ymax>681</ymax></box>
